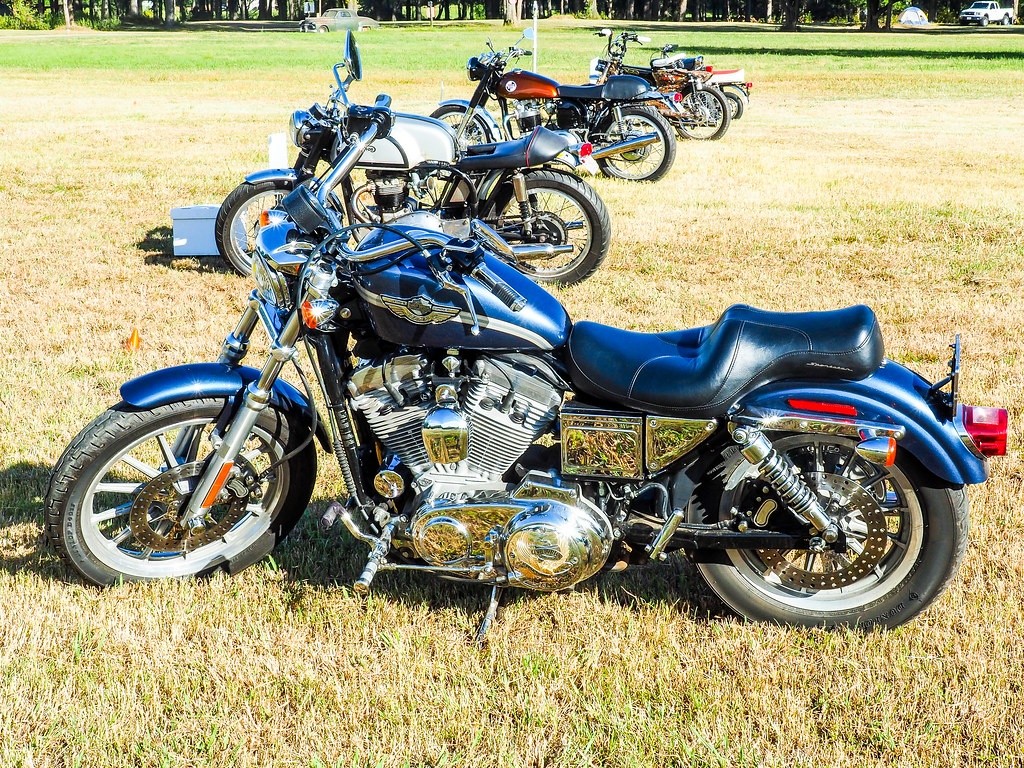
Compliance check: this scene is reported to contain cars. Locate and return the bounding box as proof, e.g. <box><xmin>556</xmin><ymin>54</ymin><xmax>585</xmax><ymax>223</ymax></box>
<box><xmin>298</xmin><ymin>9</ymin><xmax>380</xmax><ymax>33</ymax></box>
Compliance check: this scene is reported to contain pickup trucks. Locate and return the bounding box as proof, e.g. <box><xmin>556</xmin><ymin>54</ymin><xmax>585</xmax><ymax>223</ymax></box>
<box><xmin>959</xmin><ymin>1</ymin><xmax>1014</xmax><ymax>27</ymax></box>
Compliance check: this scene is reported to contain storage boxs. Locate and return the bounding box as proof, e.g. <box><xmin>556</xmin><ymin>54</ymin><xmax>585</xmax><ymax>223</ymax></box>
<box><xmin>174</xmin><ymin>205</ymin><xmax>247</xmax><ymax>256</ymax></box>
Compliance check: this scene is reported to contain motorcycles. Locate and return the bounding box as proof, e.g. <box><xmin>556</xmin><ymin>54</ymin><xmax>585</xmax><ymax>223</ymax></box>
<box><xmin>427</xmin><ymin>28</ymin><xmax>677</xmax><ymax>185</ymax></box>
<box><xmin>579</xmin><ymin>27</ymin><xmax>753</xmax><ymax>143</ymax></box>
<box><xmin>45</xmin><ymin>30</ymin><xmax>1009</xmax><ymax>635</ymax></box>
<box><xmin>212</xmin><ymin>29</ymin><xmax>611</xmax><ymax>290</ymax></box>
